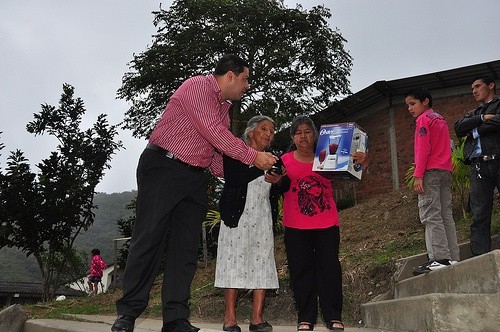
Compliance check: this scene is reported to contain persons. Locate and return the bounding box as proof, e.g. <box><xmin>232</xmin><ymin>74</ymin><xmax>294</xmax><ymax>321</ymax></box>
<box><xmin>111</xmin><ymin>54</ymin><xmax>279</xmax><ymax>332</ymax></box>
<box><xmin>87</xmin><ymin>248</ymin><xmax>108</xmax><ymax>299</ymax></box>
<box><xmin>280</xmin><ymin>117</ymin><xmax>370</xmax><ymax>332</ymax></box>
<box><xmin>454</xmin><ymin>76</ymin><xmax>500</xmax><ymax>255</ymax></box>
<box><xmin>214</xmin><ymin>116</ymin><xmax>291</xmax><ymax>332</ymax></box>
<box><xmin>404</xmin><ymin>86</ymin><xmax>460</xmax><ymax>277</ymax></box>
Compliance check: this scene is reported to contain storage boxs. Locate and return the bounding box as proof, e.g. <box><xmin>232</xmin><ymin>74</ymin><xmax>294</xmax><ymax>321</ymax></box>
<box><xmin>312</xmin><ymin>122</ymin><xmax>369</xmax><ymax>182</ymax></box>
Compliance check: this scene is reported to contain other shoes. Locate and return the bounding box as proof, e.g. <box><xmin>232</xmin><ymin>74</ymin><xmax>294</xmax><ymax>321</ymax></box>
<box><xmin>249</xmin><ymin>321</ymin><xmax>272</xmax><ymax>332</ymax></box>
<box><xmin>223</xmin><ymin>324</ymin><xmax>241</xmax><ymax>332</ymax></box>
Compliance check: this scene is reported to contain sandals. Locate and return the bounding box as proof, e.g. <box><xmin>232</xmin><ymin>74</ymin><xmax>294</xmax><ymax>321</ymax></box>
<box><xmin>297</xmin><ymin>321</ymin><xmax>314</xmax><ymax>331</ymax></box>
<box><xmin>327</xmin><ymin>320</ymin><xmax>344</xmax><ymax>330</ymax></box>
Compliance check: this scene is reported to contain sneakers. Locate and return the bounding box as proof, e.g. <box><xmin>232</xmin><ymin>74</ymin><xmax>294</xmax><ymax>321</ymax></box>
<box><xmin>413</xmin><ymin>260</ymin><xmax>458</xmax><ymax>276</ymax></box>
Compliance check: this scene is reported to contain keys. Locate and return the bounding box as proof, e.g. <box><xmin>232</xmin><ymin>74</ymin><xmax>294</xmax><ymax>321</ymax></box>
<box><xmin>475</xmin><ymin>165</ymin><xmax>482</xmax><ymax>178</ymax></box>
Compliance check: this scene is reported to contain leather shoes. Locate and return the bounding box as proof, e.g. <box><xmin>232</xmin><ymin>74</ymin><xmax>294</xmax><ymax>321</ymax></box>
<box><xmin>161</xmin><ymin>319</ymin><xmax>200</xmax><ymax>332</ymax></box>
<box><xmin>111</xmin><ymin>315</ymin><xmax>135</xmax><ymax>332</ymax></box>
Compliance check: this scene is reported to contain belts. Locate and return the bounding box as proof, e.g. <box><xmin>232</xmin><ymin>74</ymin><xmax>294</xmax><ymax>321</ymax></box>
<box><xmin>472</xmin><ymin>154</ymin><xmax>500</xmax><ymax>162</ymax></box>
<box><xmin>146</xmin><ymin>143</ymin><xmax>209</xmax><ymax>172</ymax></box>
